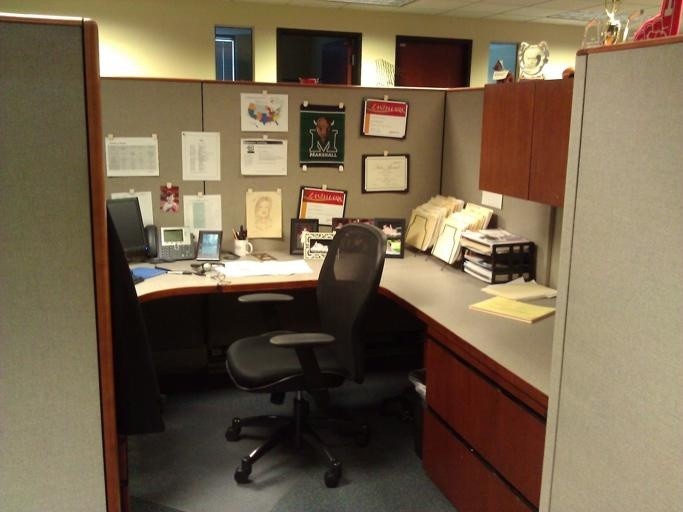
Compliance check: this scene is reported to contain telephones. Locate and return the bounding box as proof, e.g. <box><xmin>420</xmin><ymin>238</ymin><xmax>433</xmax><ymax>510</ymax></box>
<box><xmin>145</xmin><ymin>226</ymin><xmax>195</xmax><ymax>260</ymax></box>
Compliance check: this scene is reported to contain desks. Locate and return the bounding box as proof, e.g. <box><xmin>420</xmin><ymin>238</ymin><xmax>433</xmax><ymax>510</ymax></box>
<box><xmin>132</xmin><ymin>248</ymin><xmax>557</xmax><ymax>512</ymax></box>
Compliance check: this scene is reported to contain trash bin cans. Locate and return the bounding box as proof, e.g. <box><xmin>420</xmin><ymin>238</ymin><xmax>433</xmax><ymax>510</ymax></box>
<box><xmin>409</xmin><ymin>366</ymin><xmax>426</xmax><ymax>459</ymax></box>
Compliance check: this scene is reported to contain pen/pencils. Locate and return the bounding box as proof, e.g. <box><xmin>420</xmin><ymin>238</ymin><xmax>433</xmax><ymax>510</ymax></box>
<box><xmin>233</xmin><ymin>225</ymin><xmax>247</xmax><ymax>239</ymax></box>
<box><xmin>165</xmin><ymin>271</ymin><xmax>192</xmax><ymax>274</ymax></box>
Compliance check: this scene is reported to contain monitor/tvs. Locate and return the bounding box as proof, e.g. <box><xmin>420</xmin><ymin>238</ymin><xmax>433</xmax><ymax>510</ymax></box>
<box><xmin>106</xmin><ymin>196</ymin><xmax>148</xmax><ymax>254</ymax></box>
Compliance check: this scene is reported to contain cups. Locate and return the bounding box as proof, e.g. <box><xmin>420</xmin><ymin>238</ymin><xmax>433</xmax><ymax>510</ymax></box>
<box><xmin>233</xmin><ymin>239</ymin><xmax>253</xmax><ymax>258</ymax></box>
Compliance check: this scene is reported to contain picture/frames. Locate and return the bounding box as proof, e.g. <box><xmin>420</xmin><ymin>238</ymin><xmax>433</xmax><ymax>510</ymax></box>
<box><xmin>196</xmin><ymin>230</ymin><xmax>223</xmax><ymax>261</ymax></box>
<box><xmin>303</xmin><ymin>232</ymin><xmax>339</xmax><ymax>262</ymax></box>
<box><xmin>373</xmin><ymin>218</ymin><xmax>405</xmax><ymax>258</ymax></box>
<box><xmin>290</xmin><ymin>218</ymin><xmax>319</xmax><ymax>254</ymax></box>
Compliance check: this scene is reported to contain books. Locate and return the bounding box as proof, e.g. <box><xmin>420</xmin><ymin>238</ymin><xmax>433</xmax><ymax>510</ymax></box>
<box><xmin>459</xmin><ymin>225</ymin><xmax>533</xmax><ymax>285</ymax></box>
<box><xmin>467</xmin><ymin>296</ymin><xmax>556</xmax><ymax>325</ymax></box>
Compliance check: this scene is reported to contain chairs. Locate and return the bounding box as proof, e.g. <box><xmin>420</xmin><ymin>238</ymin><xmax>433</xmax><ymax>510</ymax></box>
<box><xmin>224</xmin><ymin>223</ymin><xmax>387</xmax><ymax>489</ymax></box>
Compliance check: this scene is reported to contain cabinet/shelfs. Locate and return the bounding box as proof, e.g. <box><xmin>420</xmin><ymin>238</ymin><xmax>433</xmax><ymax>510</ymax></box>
<box><xmin>479</xmin><ymin>78</ymin><xmax>575</xmax><ymax>208</ymax></box>
